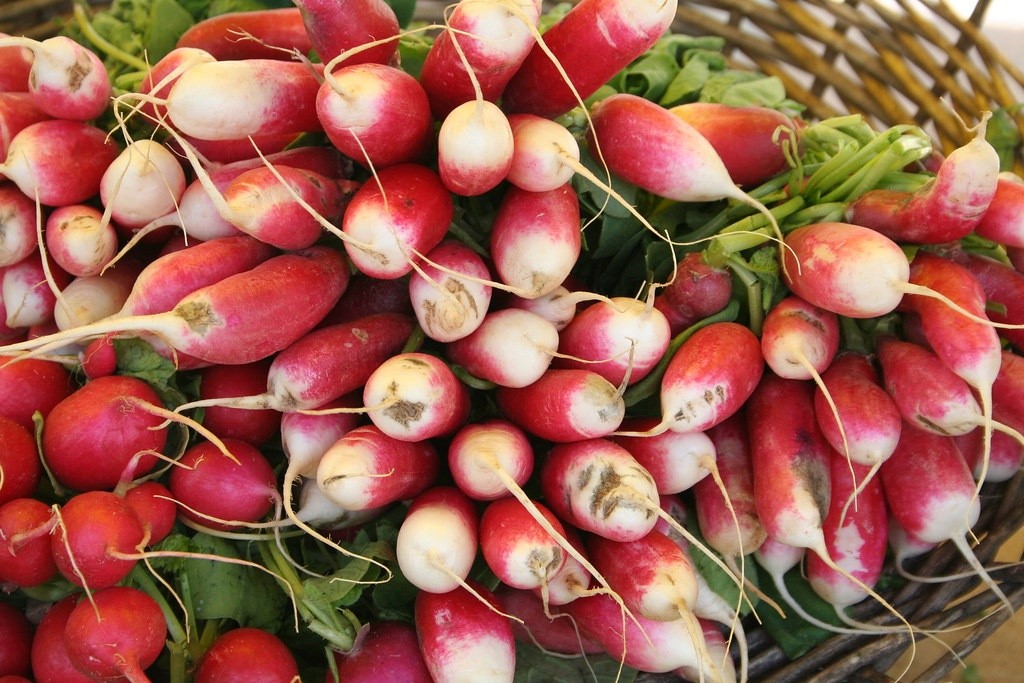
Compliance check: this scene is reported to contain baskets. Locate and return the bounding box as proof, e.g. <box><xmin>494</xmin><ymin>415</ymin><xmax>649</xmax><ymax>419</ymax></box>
<box><xmin>0</xmin><ymin>0</ymin><xmax>1024</xmax><ymax>683</ymax></box>
